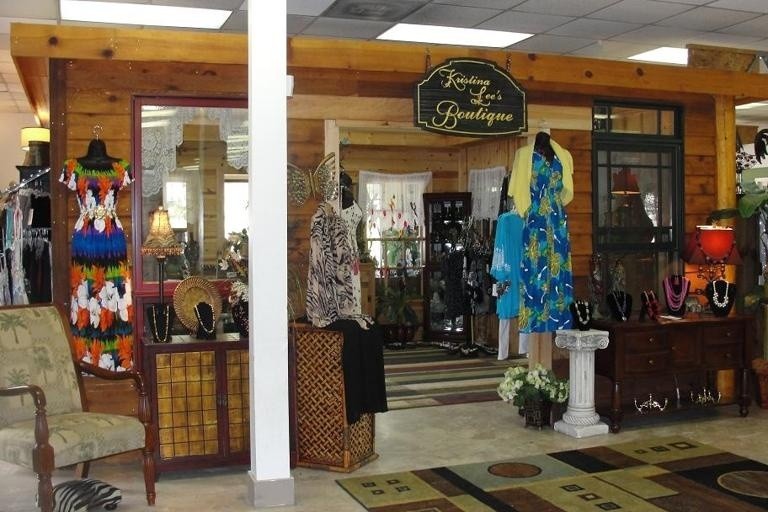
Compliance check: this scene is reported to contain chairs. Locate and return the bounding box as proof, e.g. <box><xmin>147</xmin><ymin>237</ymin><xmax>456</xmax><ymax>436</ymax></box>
<box><xmin>0</xmin><ymin>304</ymin><xmax>158</xmax><ymax>511</ymax></box>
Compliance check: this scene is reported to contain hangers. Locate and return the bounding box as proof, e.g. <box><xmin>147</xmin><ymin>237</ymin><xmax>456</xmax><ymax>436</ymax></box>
<box><xmin>11</xmin><ymin>227</ymin><xmax>50</xmax><ymax>250</ymax></box>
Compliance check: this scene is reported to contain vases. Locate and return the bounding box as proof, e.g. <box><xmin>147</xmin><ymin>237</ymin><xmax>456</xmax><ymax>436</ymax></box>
<box><xmin>230</xmin><ymin>298</ymin><xmax>248</xmax><ymax>337</ymax></box>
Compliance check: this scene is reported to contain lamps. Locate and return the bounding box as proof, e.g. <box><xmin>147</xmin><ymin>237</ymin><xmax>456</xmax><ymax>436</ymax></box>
<box><xmin>139</xmin><ymin>204</ymin><xmax>184</xmax><ymax>304</ymax></box>
<box><xmin>20</xmin><ymin>127</ymin><xmax>50</xmax><ymax>152</ymax></box>
<box><xmin>611</xmin><ymin>167</ymin><xmax>640</xmax><ymax>195</ymax></box>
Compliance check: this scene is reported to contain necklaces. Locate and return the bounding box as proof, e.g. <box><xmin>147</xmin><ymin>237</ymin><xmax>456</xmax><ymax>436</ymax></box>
<box><xmin>575</xmin><ymin>300</ymin><xmax>590</xmax><ymax>325</ymax></box>
<box><xmin>663</xmin><ymin>276</ymin><xmax>689</xmax><ymax>312</ymax></box>
<box><xmin>152</xmin><ymin>303</ymin><xmax>169</xmax><ymax>342</ymax></box>
<box><xmin>643</xmin><ymin>290</ymin><xmax>656</xmax><ymax>302</ymax></box>
<box><xmin>712</xmin><ymin>279</ymin><xmax>729</xmax><ymax>309</ymax></box>
<box><xmin>612</xmin><ymin>290</ymin><xmax>628</xmax><ymax>321</ymax></box>
<box><xmin>194</xmin><ymin>303</ymin><xmax>215</xmax><ymax>333</ymax></box>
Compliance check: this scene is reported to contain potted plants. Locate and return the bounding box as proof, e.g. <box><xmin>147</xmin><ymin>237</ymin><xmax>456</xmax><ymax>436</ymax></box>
<box><xmin>709</xmin><ymin>127</ymin><xmax>768</xmax><ymax>410</ymax></box>
<box><xmin>368</xmin><ymin>227</ymin><xmax>423</xmax><ymax>346</ymax></box>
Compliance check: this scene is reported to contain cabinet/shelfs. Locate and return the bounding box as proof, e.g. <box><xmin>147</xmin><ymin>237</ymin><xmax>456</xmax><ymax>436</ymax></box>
<box><xmin>422</xmin><ymin>191</ymin><xmax>473</xmax><ymax>344</ymax></box>
<box><xmin>359</xmin><ymin>262</ymin><xmax>376</xmax><ymax>319</ymax></box>
<box><xmin>590</xmin><ymin>312</ymin><xmax>756</xmax><ymax>433</ymax></box>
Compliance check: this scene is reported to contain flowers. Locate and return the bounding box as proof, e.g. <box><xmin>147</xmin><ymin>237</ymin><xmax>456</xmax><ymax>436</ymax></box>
<box><xmin>496</xmin><ymin>361</ymin><xmax>570</xmax><ymax>410</ymax></box>
<box><xmin>215</xmin><ymin>228</ymin><xmax>248</xmax><ymax>310</ymax></box>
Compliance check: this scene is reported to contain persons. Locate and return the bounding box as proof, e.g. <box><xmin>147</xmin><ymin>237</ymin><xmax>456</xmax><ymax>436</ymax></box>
<box><xmin>507</xmin><ymin>133</ymin><xmax>574</xmax><ymax>373</ymax></box>
<box><xmin>311</xmin><ymin>172</ymin><xmax>363</xmax><ymax>327</ymax></box>
<box><xmin>60</xmin><ymin>141</ymin><xmax>136</xmax><ymax>377</ymax></box>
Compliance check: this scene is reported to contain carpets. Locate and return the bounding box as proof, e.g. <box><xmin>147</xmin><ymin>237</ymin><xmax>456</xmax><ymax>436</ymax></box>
<box><xmin>334</xmin><ymin>435</ymin><xmax>768</xmax><ymax>512</ymax></box>
<box><xmin>381</xmin><ymin>339</ymin><xmax>530</xmax><ymax>409</ymax></box>
